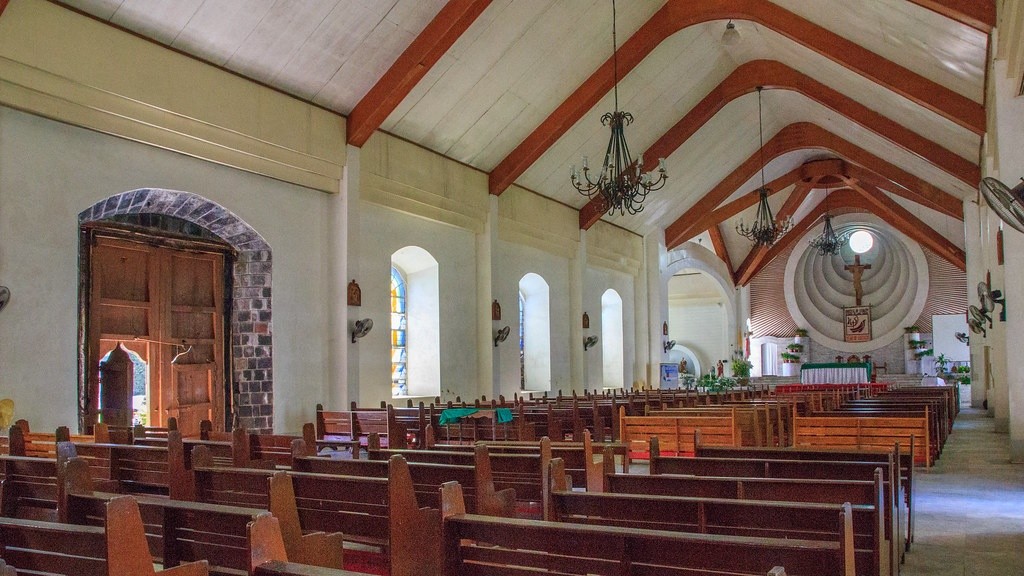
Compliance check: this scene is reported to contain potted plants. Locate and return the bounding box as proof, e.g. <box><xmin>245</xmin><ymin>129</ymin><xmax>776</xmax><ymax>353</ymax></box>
<box><xmin>781</xmin><ymin>353</ymin><xmax>802</xmax><ymax>364</ymax></box>
<box><xmin>787</xmin><ymin>344</ymin><xmax>803</xmax><ymax>352</ymax></box>
<box><xmin>934</xmin><ymin>354</ymin><xmax>970</xmax><ymax>385</ymax></box>
<box><xmin>734</xmin><ymin>358</ymin><xmax>753</xmax><ymax>385</ymax></box>
<box><xmin>903</xmin><ymin>326</ymin><xmax>919</xmax><ymax>333</ymax></box>
<box><xmin>915</xmin><ymin>349</ymin><xmax>933</xmax><ymax>360</ymax></box>
<box><xmin>794</xmin><ymin>329</ymin><xmax>807</xmax><ymax>337</ymax></box>
<box><xmin>909</xmin><ymin>339</ymin><xmax>927</xmax><ymax>349</ymax></box>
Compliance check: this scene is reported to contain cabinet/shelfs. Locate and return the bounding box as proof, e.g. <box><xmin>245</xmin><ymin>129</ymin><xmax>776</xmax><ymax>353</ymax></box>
<box><xmin>904</xmin><ymin>332</ymin><xmax>936</xmax><ymax>374</ymax></box>
<box><xmin>782</xmin><ymin>338</ymin><xmax>809</xmax><ymax>376</ymax></box>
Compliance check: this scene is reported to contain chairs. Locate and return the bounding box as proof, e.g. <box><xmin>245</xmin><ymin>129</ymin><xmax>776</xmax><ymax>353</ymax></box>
<box><xmin>835</xmin><ymin>355</ymin><xmax>872</xmax><ymax>363</ymax></box>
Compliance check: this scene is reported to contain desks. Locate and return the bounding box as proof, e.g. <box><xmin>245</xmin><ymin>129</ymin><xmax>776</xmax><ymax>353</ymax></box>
<box><xmin>800</xmin><ymin>362</ymin><xmax>871</xmax><ymax>383</ymax></box>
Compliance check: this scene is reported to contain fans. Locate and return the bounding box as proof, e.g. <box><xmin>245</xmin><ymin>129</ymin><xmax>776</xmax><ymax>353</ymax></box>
<box><xmin>585</xmin><ymin>335</ymin><xmax>599</xmax><ymax>351</ymax></box>
<box><xmin>495</xmin><ymin>326</ymin><xmax>510</xmax><ymax>347</ymax></box>
<box><xmin>0</xmin><ymin>285</ymin><xmax>11</xmax><ymax>312</ymax></box>
<box><xmin>664</xmin><ymin>340</ymin><xmax>676</xmax><ymax>353</ymax></box>
<box><xmin>977</xmin><ymin>281</ymin><xmax>1005</xmax><ymax>322</ymax></box>
<box><xmin>969</xmin><ymin>318</ymin><xmax>987</xmax><ymax>337</ymax></box>
<box><xmin>955</xmin><ymin>331</ymin><xmax>969</xmax><ymax>346</ymax></box>
<box><xmin>981</xmin><ymin>177</ymin><xmax>1024</xmax><ymax>234</ymax></box>
<box><xmin>352</xmin><ymin>318</ymin><xmax>374</xmax><ymax>343</ymax></box>
<box><xmin>969</xmin><ymin>305</ymin><xmax>992</xmax><ymax>329</ymax></box>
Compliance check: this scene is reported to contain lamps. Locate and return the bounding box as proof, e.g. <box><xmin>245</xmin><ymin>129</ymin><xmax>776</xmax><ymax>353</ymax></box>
<box><xmin>567</xmin><ymin>0</ymin><xmax>669</xmax><ymax>216</ymax></box>
<box><xmin>721</xmin><ymin>19</ymin><xmax>742</xmax><ymax>46</ymax></box>
<box><xmin>734</xmin><ymin>86</ymin><xmax>795</xmax><ymax>248</ymax></box>
<box><xmin>808</xmin><ymin>179</ymin><xmax>850</xmax><ymax>258</ymax></box>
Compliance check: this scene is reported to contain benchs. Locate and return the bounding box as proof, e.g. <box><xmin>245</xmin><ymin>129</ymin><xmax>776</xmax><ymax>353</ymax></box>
<box><xmin>0</xmin><ymin>381</ymin><xmax>959</xmax><ymax>576</ymax></box>
